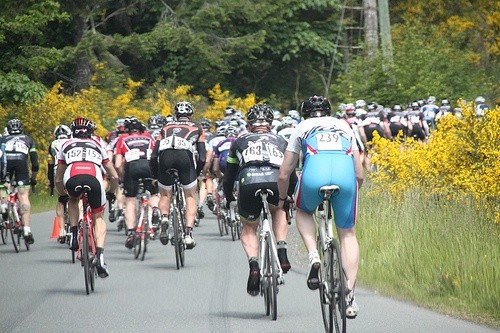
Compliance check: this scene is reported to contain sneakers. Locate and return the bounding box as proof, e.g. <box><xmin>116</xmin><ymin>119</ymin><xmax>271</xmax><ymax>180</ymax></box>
<box><xmin>158</xmin><ymin>217</ymin><xmax>170</xmax><ymax>246</ymax></box>
<box><xmin>306</xmin><ymin>250</ymin><xmax>320</xmax><ymax>290</ymax></box>
<box><xmin>69</xmin><ymin>227</ymin><xmax>79</xmax><ymax>251</ymax></box>
<box><xmin>56</xmin><ymin>228</ymin><xmax>68</xmax><ymax>243</ymax></box>
<box><xmin>206</xmin><ymin>194</ymin><xmax>215</xmax><ymax>211</ymax></box>
<box><xmin>183</xmin><ymin>226</ymin><xmax>197</xmax><ymax>248</ymax></box>
<box><xmin>22</xmin><ymin>230</ymin><xmax>34</xmax><ymax>244</ymax></box>
<box><xmin>125</xmin><ymin>231</ymin><xmax>135</xmax><ymax>250</ymax></box>
<box><xmin>1</xmin><ymin>208</ymin><xmax>8</xmax><ymax>219</ymax></box>
<box><xmin>247</xmin><ymin>262</ymin><xmax>260</xmax><ymax>298</ymax></box>
<box><xmin>277</xmin><ymin>249</ymin><xmax>291</xmax><ymax>275</ymax></box>
<box><xmin>116</xmin><ymin>209</ymin><xmax>125</xmax><ymax>232</ymax></box>
<box><xmin>152</xmin><ymin>206</ymin><xmax>161</xmax><ymax>224</ymax></box>
<box><xmin>96</xmin><ymin>253</ymin><xmax>110</xmax><ymax>279</ymax></box>
<box><xmin>344</xmin><ymin>288</ymin><xmax>360</xmax><ymax>319</ymax></box>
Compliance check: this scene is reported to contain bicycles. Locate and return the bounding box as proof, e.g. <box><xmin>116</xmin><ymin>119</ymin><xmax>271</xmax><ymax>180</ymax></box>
<box><xmin>74</xmin><ymin>182</ymin><xmax>99</xmax><ymax>295</ymax></box>
<box><xmin>166</xmin><ymin>165</ymin><xmax>188</xmax><ymax>267</ymax></box>
<box><xmin>59</xmin><ymin>193</ymin><xmax>74</xmax><ymax>250</ymax></box>
<box><xmin>129</xmin><ymin>178</ymin><xmax>150</xmax><ymax>258</ymax></box>
<box><xmin>312</xmin><ymin>185</ymin><xmax>347</xmax><ymax>333</ymax></box>
<box><xmin>215</xmin><ymin>170</ymin><xmax>244</xmax><ymax>240</ymax></box>
<box><xmin>255</xmin><ymin>187</ymin><xmax>279</xmax><ymax>320</ymax></box>
<box><xmin>113</xmin><ymin>194</ymin><xmax>205</xmax><ymax>236</ymax></box>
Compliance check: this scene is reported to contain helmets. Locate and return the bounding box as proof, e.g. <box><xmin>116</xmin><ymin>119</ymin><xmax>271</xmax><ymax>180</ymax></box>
<box><xmin>245</xmin><ymin>103</ymin><xmax>274</xmax><ymax>129</ymax></box>
<box><xmin>115</xmin><ymin>113</ymin><xmax>167</xmax><ymax>131</ymax></box>
<box><xmin>300</xmin><ymin>94</ymin><xmax>333</xmax><ymax>118</ymax></box>
<box><xmin>272</xmin><ymin>110</ymin><xmax>301</xmax><ymax>128</ymax></box>
<box><xmin>339</xmin><ymin>96</ymin><xmax>486</xmax><ymax>118</ymax></box>
<box><xmin>174</xmin><ymin>101</ymin><xmax>194</xmax><ymax>117</ymax></box>
<box><xmin>69</xmin><ymin>118</ymin><xmax>98</xmax><ymax>138</ymax></box>
<box><xmin>6</xmin><ymin>119</ymin><xmax>23</xmax><ymax>135</ymax></box>
<box><xmin>199</xmin><ymin>107</ymin><xmax>241</xmax><ymax>136</ymax></box>
<box><xmin>52</xmin><ymin>124</ymin><xmax>71</xmax><ymax>139</ymax></box>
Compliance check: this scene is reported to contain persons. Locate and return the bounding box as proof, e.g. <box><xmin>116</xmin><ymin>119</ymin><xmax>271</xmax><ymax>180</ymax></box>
<box><xmin>221</xmin><ymin>102</ymin><xmax>298</xmax><ymax>298</ymax></box>
<box><xmin>0</xmin><ymin>94</ymin><xmax>489</xmax><ymax>223</ymax></box>
<box><xmin>277</xmin><ymin>95</ymin><xmax>365</xmax><ymax>318</ymax></box>
<box><xmin>54</xmin><ymin>119</ymin><xmax>119</xmax><ymax>278</ymax></box>
<box><xmin>149</xmin><ymin>101</ymin><xmax>207</xmax><ymax>248</ymax></box>
<box><xmin>0</xmin><ymin>118</ymin><xmax>40</xmax><ymax>246</ymax></box>
<box><xmin>115</xmin><ymin>117</ymin><xmax>159</xmax><ymax>250</ymax></box>
